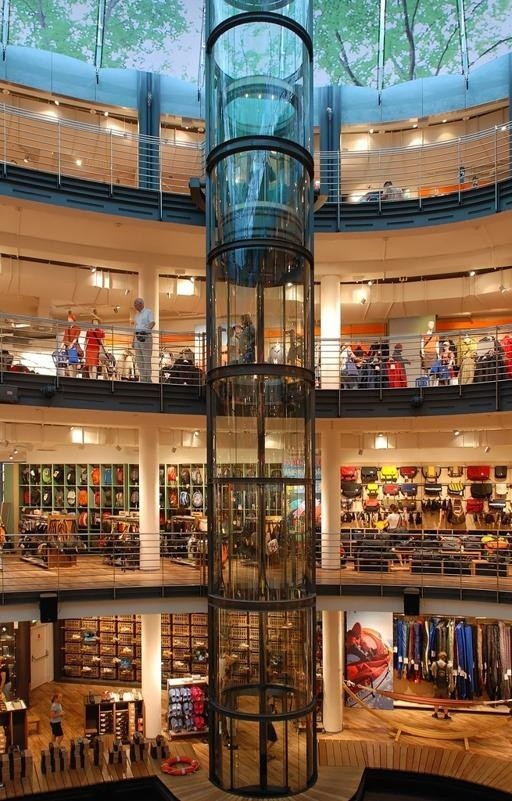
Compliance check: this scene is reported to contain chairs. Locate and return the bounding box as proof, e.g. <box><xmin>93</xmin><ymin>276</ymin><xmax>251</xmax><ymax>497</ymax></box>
<box><xmin>52</xmin><ymin>350</ymin><xmax>69</xmax><ymax>374</ymax></box>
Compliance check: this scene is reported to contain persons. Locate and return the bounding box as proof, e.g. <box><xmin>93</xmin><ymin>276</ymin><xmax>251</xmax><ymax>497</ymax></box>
<box><xmin>49</xmin><ymin>693</ymin><xmax>66</xmax><ymax>753</ymax></box>
<box><xmin>62</xmin><ymin>311</ymin><xmax>80</xmax><ymax>378</ymax></box>
<box><xmin>346</xmin><ymin>622</ymin><xmax>375</xmax><ymax>664</ymax></box>
<box><xmin>0</xmin><ymin>350</ymin><xmax>13</xmax><ymax>371</ymax></box>
<box><xmin>219</xmin><ymin>671</ymin><xmax>239</xmax><ymax>752</ymax></box>
<box><xmin>0</xmin><ymin>751</ymin><xmax>7</xmax><ymax>790</ymax></box>
<box><xmin>314</xmin><ymin>181</ymin><xmax>320</xmax><ymax>200</ymax></box>
<box><xmin>240</xmin><ymin>146</ymin><xmax>277</xmax><ymax>201</ymax></box>
<box><xmin>228</xmin><ymin>314</ymin><xmax>256</xmax><ymax>380</ymax></box>
<box><xmin>431</xmin><ymin>651</ymin><xmax>454</xmax><ymax>720</ymax></box>
<box><xmin>339</xmin><ymin>342</ymin><xmax>411</xmax><ymax>387</ymax></box>
<box><xmin>0</xmin><ymin>515</ymin><xmax>7</xmax><ymax>572</ymax></box>
<box><xmin>132</xmin><ymin>297</ymin><xmax>156</xmax><ymax>382</ymax></box>
<box><xmin>237</xmin><ymin>514</ymin><xmax>296</xmax><ymax>570</ymax></box>
<box><xmin>83</xmin><ymin>317</ymin><xmax>105</xmax><ymax>379</ymax></box>
<box><xmin>438</xmin><ymin>341</ymin><xmax>456</xmax><ymax>385</ymax></box>
<box><xmin>361</xmin><ymin>180</ymin><xmax>404</xmax><ymax>201</ymax></box>
<box><xmin>384</xmin><ymin>504</ymin><xmax>404</xmax><ymax>568</ymax></box>
<box><xmin>266</xmin><ymin>694</ymin><xmax>280</xmax><ymax>759</ymax></box>
<box><xmin>0</xmin><ymin>656</ymin><xmax>12</xmax><ymax>702</ymax></box>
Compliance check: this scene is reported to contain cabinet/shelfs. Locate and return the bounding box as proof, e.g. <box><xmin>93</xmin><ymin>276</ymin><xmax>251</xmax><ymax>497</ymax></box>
<box><xmin>340</xmin><ymin>525</ymin><xmax>512</xmax><ymax>579</ymax></box>
<box><xmin>3</xmin><ymin>460</ymin><xmax>283</xmax><ymax>559</ymax></box>
<box><xmin>81</xmin><ymin>693</ymin><xmax>146</xmax><ymax>747</ymax></box>
<box><xmin>166</xmin><ymin>678</ymin><xmax>207</xmax><ymax>742</ymax></box>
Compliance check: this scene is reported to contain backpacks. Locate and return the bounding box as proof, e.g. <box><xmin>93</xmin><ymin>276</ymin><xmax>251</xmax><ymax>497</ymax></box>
<box><xmin>435</xmin><ymin>662</ymin><xmax>450</xmax><ymax>688</ymax></box>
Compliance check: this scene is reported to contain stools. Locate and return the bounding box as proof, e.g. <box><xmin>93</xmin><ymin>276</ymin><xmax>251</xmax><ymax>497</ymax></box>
<box><xmin>28</xmin><ymin>716</ymin><xmax>41</xmax><ymax>734</ymax></box>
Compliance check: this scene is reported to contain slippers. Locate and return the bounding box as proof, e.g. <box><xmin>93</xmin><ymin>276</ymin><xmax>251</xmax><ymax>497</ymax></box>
<box><xmin>168</xmin><ymin>686</ymin><xmax>205</xmax><ymax>733</ymax></box>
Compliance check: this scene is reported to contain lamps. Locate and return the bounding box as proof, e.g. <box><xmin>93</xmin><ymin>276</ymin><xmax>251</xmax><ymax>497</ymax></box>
<box><xmin>24</xmin><ymin>153</ymin><xmax>30</xmax><ymax>163</ymax></box>
<box><xmin>114</xmin><ymin>307</ymin><xmax>119</xmax><ymax>314</ymax></box>
<box><xmin>368</xmin><ymin>280</ymin><xmax>374</xmax><ymax>286</ymax></box>
<box><xmin>360</xmin><ymin>299</ymin><xmax>365</xmax><ymax>305</ymax></box>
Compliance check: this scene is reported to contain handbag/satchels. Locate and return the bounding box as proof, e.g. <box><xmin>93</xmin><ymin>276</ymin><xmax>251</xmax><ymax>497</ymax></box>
<box><xmin>167</xmin><ymin>465</ymin><xmax>203</xmax><ymax>508</ymax></box>
<box><xmin>346</xmin><ymin>362</ymin><xmax>358</xmax><ymax>376</ymax></box>
<box><xmin>340</xmin><ymin>464</ymin><xmax>512</xmax><ymax>524</ymax></box>
<box><xmin>68</xmin><ymin>343</ymin><xmax>84</xmax><ymax>363</ymax></box>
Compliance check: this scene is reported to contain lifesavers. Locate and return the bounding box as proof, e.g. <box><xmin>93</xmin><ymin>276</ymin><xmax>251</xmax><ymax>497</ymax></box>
<box><xmin>160</xmin><ymin>755</ymin><xmax>200</xmax><ymax>775</ymax></box>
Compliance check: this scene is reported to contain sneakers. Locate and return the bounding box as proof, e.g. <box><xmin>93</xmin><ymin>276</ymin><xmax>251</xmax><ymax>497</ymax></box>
<box><xmin>431</xmin><ymin>713</ymin><xmax>451</xmax><ymax>720</ymax></box>
